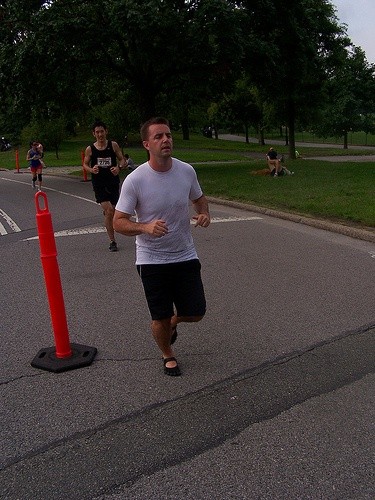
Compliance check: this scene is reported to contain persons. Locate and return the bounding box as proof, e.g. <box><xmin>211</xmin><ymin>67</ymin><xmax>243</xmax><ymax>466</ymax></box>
<box><xmin>83</xmin><ymin>123</ymin><xmax>125</xmax><ymax>251</ymax></box>
<box><xmin>0</xmin><ymin>136</ymin><xmax>11</xmax><ymax>152</ymax></box>
<box><xmin>112</xmin><ymin>118</ymin><xmax>210</xmax><ymax>376</ymax></box>
<box><xmin>26</xmin><ymin>141</ymin><xmax>45</xmax><ymax>192</ymax></box>
<box><xmin>249</xmin><ymin>145</ymin><xmax>303</xmax><ymax>177</ymax></box>
<box><xmin>123</xmin><ymin>154</ymin><xmax>134</xmax><ymax>170</ymax></box>
<box><xmin>200</xmin><ymin>126</ymin><xmax>213</xmax><ymax>138</ymax></box>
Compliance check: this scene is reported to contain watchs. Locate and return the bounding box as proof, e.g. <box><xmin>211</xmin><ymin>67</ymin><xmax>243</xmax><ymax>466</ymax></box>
<box><xmin>116</xmin><ymin>164</ymin><xmax>122</xmax><ymax>170</ymax></box>
